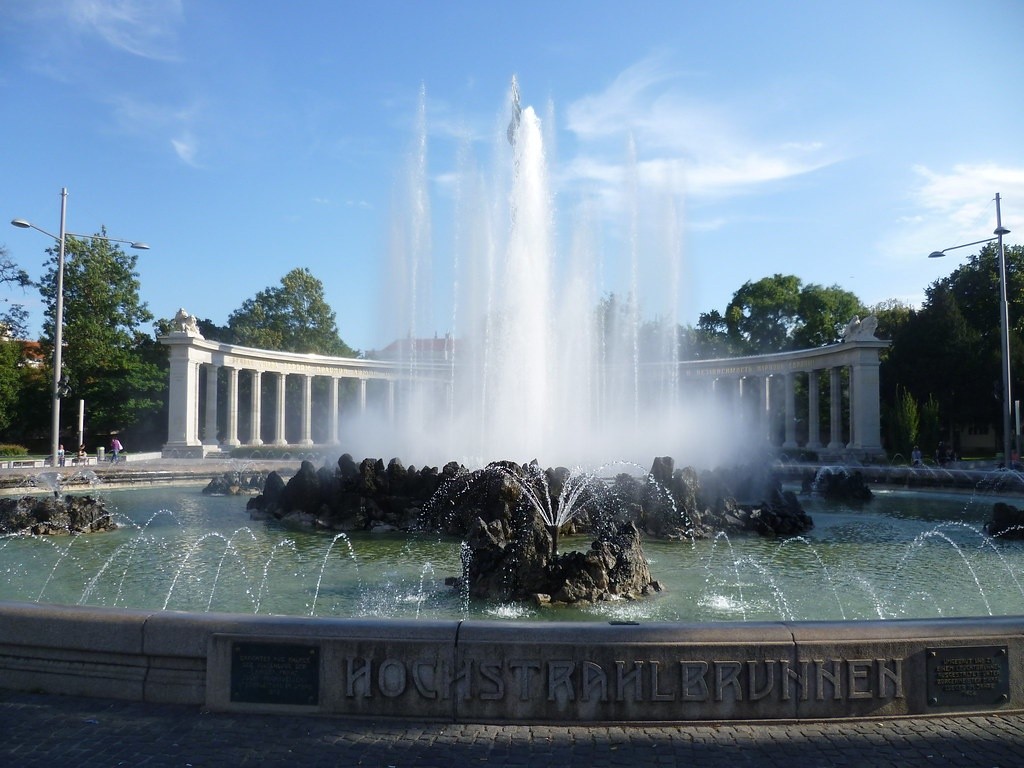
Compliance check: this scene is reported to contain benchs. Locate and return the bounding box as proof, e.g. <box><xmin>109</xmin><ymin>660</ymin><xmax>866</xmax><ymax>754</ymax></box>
<box><xmin>0</xmin><ymin>462</ymin><xmax>12</xmax><ymax>469</ymax></box>
<box><xmin>72</xmin><ymin>458</ymin><xmax>89</xmax><ymax>466</ymax></box>
<box><xmin>13</xmin><ymin>461</ymin><xmax>35</xmax><ymax>469</ymax></box>
<box><xmin>44</xmin><ymin>458</ymin><xmax>65</xmax><ymax>468</ymax></box>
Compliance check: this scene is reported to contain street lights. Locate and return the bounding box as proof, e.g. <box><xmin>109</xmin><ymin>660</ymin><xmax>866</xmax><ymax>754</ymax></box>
<box><xmin>11</xmin><ymin>219</ymin><xmax>150</xmax><ymax>468</ymax></box>
<box><xmin>928</xmin><ymin>234</ymin><xmax>1012</xmax><ymax>470</ymax></box>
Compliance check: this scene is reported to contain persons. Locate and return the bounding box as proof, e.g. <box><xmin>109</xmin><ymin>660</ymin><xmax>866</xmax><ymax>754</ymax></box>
<box><xmin>110</xmin><ymin>437</ymin><xmax>123</xmax><ymax>464</ymax></box>
<box><xmin>58</xmin><ymin>445</ymin><xmax>65</xmax><ymax>467</ymax></box>
<box><xmin>175</xmin><ymin>308</ymin><xmax>198</xmax><ymax>332</ymax></box>
<box><xmin>935</xmin><ymin>441</ymin><xmax>945</xmax><ymax>469</ymax></box>
<box><xmin>844</xmin><ymin>310</ymin><xmax>878</xmax><ymax>339</ymax></box>
<box><xmin>996</xmin><ymin>449</ymin><xmax>1020</xmax><ymax>471</ymax></box>
<box><xmin>77</xmin><ymin>445</ymin><xmax>88</xmax><ymax>463</ymax></box>
<box><xmin>911</xmin><ymin>446</ymin><xmax>921</xmax><ymax>468</ymax></box>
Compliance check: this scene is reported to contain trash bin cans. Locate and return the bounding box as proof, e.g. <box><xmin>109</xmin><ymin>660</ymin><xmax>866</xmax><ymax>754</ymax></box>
<box><xmin>1011</xmin><ymin>454</ymin><xmax>1020</xmax><ymax>464</ymax></box>
<box><xmin>996</xmin><ymin>453</ymin><xmax>1005</xmax><ymax>464</ymax></box>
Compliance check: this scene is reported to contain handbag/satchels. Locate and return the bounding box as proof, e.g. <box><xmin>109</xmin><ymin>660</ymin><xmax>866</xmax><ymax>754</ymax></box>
<box><xmin>118</xmin><ymin>441</ymin><xmax>123</xmax><ymax>450</ymax></box>
